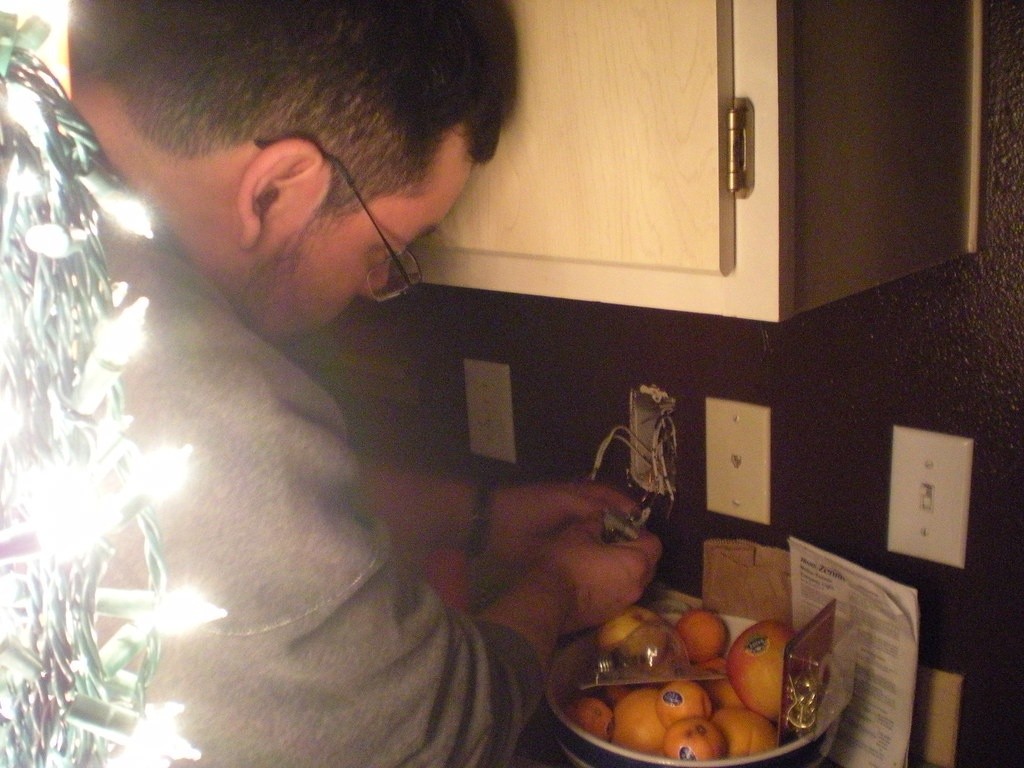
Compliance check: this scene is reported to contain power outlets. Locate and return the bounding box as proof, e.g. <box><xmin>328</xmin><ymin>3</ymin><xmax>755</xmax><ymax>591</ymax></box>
<box><xmin>704</xmin><ymin>396</ymin><xmax>773</xmax><ymax>528</ymax></box>
<box><xmin>463</xmin><ymin>359</ymin><xmax>518</xmax><ymax>465</ymax></box>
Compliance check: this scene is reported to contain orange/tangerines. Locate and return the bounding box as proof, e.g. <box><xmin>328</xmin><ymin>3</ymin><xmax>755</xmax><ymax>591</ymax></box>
<box><xmin>569</xmin><ymin>605</ymin><xmax>800</xmax><ymax>760</ymax></box>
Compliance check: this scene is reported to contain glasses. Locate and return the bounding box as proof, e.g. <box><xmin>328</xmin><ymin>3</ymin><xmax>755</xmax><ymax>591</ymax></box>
<box><xmin>255</xmin><ymin>140</ymin><xmax>422</xmax><ymax>301</ymax></box>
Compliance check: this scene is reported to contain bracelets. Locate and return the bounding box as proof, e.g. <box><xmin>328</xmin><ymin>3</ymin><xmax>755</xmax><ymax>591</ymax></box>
<box><xmin>463</xmin><ymin>467</ymin><xmax>495</xmax><ymax>557</ymax></box>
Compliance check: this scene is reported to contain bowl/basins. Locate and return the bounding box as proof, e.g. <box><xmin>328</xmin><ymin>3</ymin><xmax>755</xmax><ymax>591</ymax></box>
<box><xmin>548</xmin><ymin>610</ymin><xmax>851</xmax><ymax>768</ymax></box>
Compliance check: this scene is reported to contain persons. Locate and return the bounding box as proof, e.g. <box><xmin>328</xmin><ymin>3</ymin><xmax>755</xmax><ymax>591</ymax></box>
<box><xmin>0</xmin><ymin>0</ymin><xmax>663</xmax><ymax>767</ymax></box>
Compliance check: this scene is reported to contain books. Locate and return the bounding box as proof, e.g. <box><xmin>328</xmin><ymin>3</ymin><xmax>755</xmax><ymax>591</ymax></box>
<box><xmin>787</xmin><ymin>536</ymin><xmax>921</xmax><ymax>768</ymax></box>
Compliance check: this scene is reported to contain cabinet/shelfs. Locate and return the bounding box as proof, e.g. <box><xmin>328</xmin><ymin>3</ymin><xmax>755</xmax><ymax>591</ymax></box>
<box><xmin>399</xmin><ymin>0</ymin><xmax>967</xmax><ymax>325</ymax></box>
